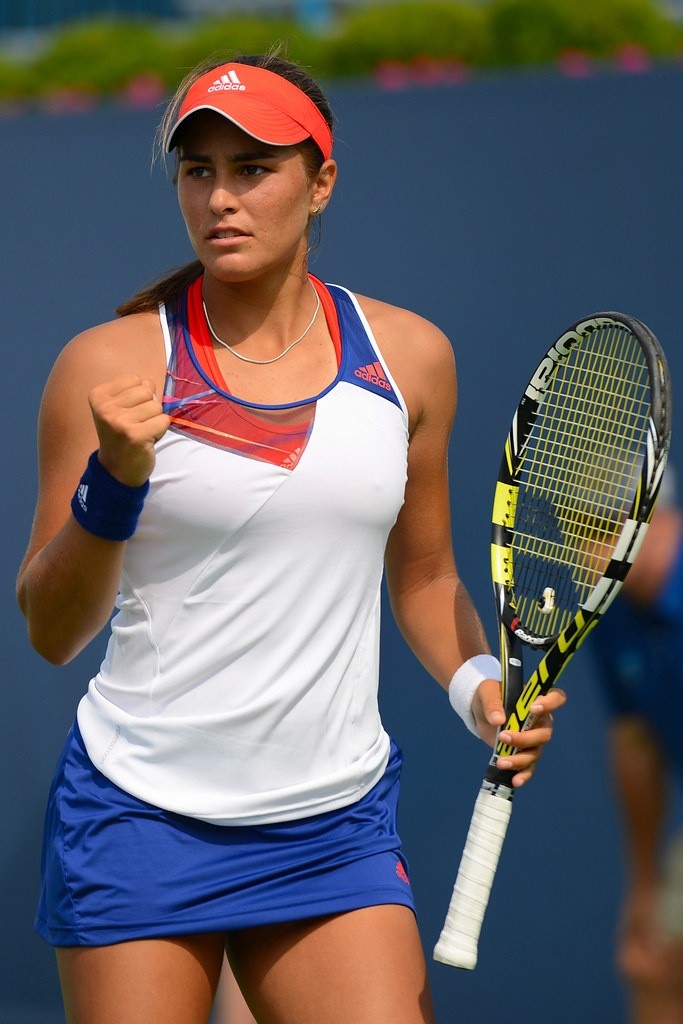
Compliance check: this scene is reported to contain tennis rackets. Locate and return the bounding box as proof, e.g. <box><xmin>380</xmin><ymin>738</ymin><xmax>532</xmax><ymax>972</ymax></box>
<box><xmin>431</xmin><ymin>310</ymin><xmax>673</xmax><ymax>974</ymax></box>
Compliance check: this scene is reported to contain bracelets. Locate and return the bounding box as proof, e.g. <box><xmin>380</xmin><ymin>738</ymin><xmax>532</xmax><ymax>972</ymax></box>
<box><xmin>448</xmin><ymin>655</ymin><xmax>503</xmax><ymax>740</ymax></box>
<box><xmin>71</xmin><ymin>452</ymin><xmax>150</xmax><ymax>542</ymax></box>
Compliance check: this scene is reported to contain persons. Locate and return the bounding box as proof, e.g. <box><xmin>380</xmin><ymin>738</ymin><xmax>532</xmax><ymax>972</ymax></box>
<box><xmin>16</xmin><ymin>40</ymin><xmax>683</xmax><ymax>1024</ymax></box>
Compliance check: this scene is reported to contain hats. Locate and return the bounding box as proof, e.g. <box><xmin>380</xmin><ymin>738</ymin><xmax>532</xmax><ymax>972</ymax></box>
<box><xmin>163</xmin><ymin>61</ymin><xmax>334</xmax><ymax>163</ymax></box>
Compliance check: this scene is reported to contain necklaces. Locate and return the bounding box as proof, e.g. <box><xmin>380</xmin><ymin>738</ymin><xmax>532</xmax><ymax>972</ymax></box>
<box><xmin>202</xmin><ymin>276</ymin><xmax>323</xmax><ymax>363</ymax></box>
